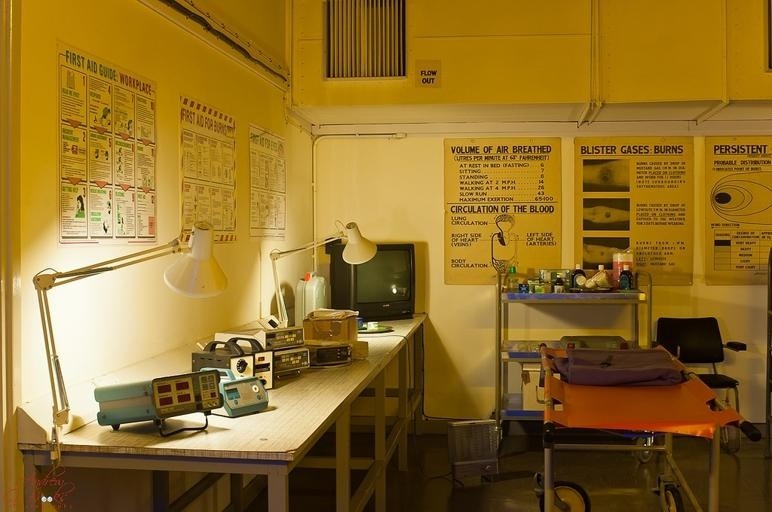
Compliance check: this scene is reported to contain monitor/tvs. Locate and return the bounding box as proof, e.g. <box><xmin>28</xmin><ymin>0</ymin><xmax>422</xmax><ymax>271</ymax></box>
<box><xmin>330</xmin><ymin>242</ymin><xmax>415</xmax><ymax>321</ymax></box>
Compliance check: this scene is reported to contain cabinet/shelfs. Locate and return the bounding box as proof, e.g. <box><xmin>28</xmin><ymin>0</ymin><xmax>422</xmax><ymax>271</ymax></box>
<box><xmin>493</xmin><ymin>268</ymin><xmax>659</xmax><ymax>462</ymax></box>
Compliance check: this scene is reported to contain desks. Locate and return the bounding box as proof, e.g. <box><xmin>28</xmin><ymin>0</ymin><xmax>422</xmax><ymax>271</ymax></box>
<box><xmin>14</xmin><ymin>312</ymin><xmax>428</xmax><ymax>511</ymax></box>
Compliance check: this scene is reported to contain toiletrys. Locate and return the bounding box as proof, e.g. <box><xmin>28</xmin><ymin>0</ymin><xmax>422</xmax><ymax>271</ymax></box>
<box><xmin>612</xmin><ymin>252</ymin><xmax>634</xmax><ymax>291</ymax></box>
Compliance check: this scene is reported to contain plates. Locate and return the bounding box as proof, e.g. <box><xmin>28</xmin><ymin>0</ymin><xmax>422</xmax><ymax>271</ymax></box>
<box><xmin>358</xmin><ymin>326</ymin><xmax>394</xmax><ymax>333</ymax></box>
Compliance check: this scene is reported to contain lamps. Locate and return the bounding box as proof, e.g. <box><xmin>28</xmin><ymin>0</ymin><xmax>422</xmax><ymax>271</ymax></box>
<box><xmin>31</xmin><ymin>221</ymin><xmax>231</xmax><ymax>436</ymax></box>
<box><xmin>269</xmin><ymin>219</ymin><xmax>379</xmax><ymax>328</ymax></box>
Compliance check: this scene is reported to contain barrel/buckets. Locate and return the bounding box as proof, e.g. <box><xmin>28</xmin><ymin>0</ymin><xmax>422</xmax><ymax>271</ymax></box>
<box><xmin>294</xmin><ymin>272</ymin><xmax>327</xmax><ymax>327</ymax></box>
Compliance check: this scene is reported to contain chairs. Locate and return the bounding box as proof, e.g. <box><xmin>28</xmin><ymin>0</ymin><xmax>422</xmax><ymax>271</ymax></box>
<box><xmin>651</xmin><ymin>313</ymin><xmax>747</xmax><ymax>454</ymax></box>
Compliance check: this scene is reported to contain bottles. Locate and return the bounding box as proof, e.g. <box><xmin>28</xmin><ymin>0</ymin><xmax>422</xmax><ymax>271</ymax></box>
<box><xmin>554</xmin><ymin>264</ymin><xmax>633</xmax><ymax>294</ymax></box>
<box><xmin>504</xmin><ymin>266</ymin><xmax>520</xmax><ymax>293</ymax></box>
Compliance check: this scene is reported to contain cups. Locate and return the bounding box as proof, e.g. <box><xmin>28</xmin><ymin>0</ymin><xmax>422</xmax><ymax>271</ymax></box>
<box><xmin>356</xmin><ymin>318</ymin><xmax>363</xmax><ymax>330</ymax></box>
<box><xmin>368</xmin><ymin>322</ymin><xmax>379</xmax><ymax>329</ymax></box>
